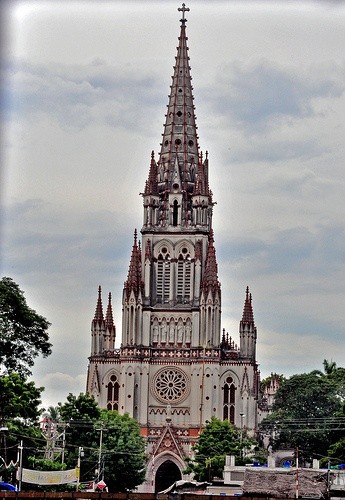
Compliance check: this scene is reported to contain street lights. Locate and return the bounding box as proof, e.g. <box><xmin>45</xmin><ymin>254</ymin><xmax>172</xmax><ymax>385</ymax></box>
<box><xmin>95</xmin><ymin>426</ymin><xmax>109</xmax><ymax>481</ymax></box>
